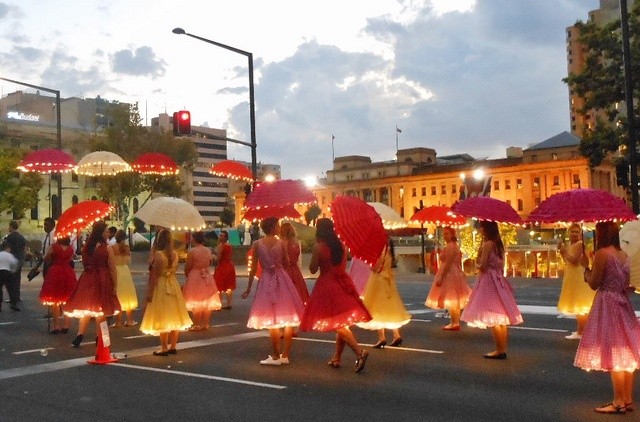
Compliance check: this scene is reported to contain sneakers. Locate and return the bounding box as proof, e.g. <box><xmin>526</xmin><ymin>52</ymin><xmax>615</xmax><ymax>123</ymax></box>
<box><xmin>483</xmin><ymin>352</ymin><xmax>506</xmax><ymax>359</ymax></box>
<box><xmin>566</xmin><ymin>332</ymin><xmax>582</xmax><ymax>339</ymax></box>
<box><xmin>442</xmin><ymin>325</ymin><xmax>460</xmax><ymax>331</ymax></box>
<box><xmin>153</xmin><ymin>349</ymin><xmax>177</xmax><ymax>357</ymax></box>
<box><xmin>260</xmin><ymin>354</ymin><xmax>289</xmax><ymax>366</ymax></box>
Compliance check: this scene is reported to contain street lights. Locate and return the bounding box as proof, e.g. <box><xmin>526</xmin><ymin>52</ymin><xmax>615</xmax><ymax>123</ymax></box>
<box><xmin>172</xmin><ymin>28</ymin><xmax>256</xmax><ymax>184</ymax></box>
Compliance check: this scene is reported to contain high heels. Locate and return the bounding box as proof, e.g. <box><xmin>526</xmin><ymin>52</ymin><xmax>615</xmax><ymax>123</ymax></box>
<box><xmin>389</xmin><ymin>337</ymin><xmax>402</xmax><ymax>348</ymax></box>
<box><xmin>373</xmin><ymin>340</ymin><xmax>386</xmax><ymax>348</ymax></box>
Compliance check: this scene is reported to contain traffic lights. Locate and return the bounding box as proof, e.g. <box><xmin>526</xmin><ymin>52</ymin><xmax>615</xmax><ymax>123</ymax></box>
<box><xmin>177</xmin><ymin>111</ymin><xmax>191</xmax><ymax>136</ymax></box>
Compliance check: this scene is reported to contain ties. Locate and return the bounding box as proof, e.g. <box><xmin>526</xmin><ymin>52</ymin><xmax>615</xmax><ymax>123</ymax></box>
<box><xmin>43</xmin><ymin>233</ymin><xmax>50</xmax><ymax>254</ymax></box>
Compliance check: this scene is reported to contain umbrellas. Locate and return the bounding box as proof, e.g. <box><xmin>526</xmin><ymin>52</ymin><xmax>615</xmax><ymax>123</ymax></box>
<box><xmin>15</xmin><ymin>148</ymin><xmax>77</xmax><ymax>200</ymax></box>
<box><xmin>365</xmin><ymin>202</ymin><xmax>408</xmax><ymax>230</ymax></box>
<box><xmin>242</xmin><ymin>178</ymin><xmax>318</xmax><ymax>209</ymax></box>
<box><xmin>451</xmin><ymin>187</ymin><xmax>524</xmax><ymax>247</ymax></box>
<box><xmin>134</xmin><ymin>195</ymin><xmax>207</xmax><ymax>250</ymax></box>
<box><xmin>524</xmin><ymin>183</ymin><xmax>637</xmax><ymax>253</ymax></box>
<box><xmin>207</xmin><ymin>158</ymin><xmax>253</xmax><ymax>192</ymax></box>
<box><xmin>54</xmin><ymin>201</ymin><xmax>115</xmax><ymax>239</ymax></box>
<box><xmin>240</xmin><ymin>205</ymin><xmax>303</xmax><ymax>224</ymax></box>
<box><xmin>409</xmin><ymin>201</ymin><xmax>466</xmax><ymax>248</ymax></box>
<box><xmin>76</xmin><ymin>151</ymin><xmax>132</xmax><ymax>191</ymax></box>
<box><xmin>618</xmin><ymin>219</ymin><xmax>640</xmax><ymax>259</ymax></box>
<box><xmin>129</xmin><ymin>153</ymin><xmax>180</xmax><ymax>175</ymax></box>
<box><xmin>328</xmin><ymin>194</ymin><xmax>387</xmax><ymax>269</ymax></box>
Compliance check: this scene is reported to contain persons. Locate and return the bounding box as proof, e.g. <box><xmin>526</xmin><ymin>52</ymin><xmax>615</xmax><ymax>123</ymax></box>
<box><xmin>0</xmin><ymin>243</ymin><xmax>20</xmax><ymax>312</ymax></box>
<box><xmin>357</xmin><ymin>234</ymin><xmax>412</xmax><ymax>348</ymax></box>
<box><xmin>76</xmin><ymin>231</ymin><xmax>84</xmax><ymax>255</ymax></box>
<box><xmin>237</xmin><ymin>220</ymin><xmax>246</xmax><ymax>245</ymax></box>
<box><xmin>211</xmin><ymin>230</ymin><xmax>236</xmax><ymax>310</ymax></box>
<box><xmin>557</xmin><ymin>223</ymin><xmax>599</xmax><ymax>340</ymax></box>
<box><xmin>241</xmin><ymin>215</ymin><xmax>303</xmax><ymax>366</ymax></box>
<box><xmin>347</xmin><ymin>252</ymin><xmax>374</xmax><ymax>298</ymax></box>
<box><xmin>579</xmin><ymin>219</ymin><xmax>640</xmax><ymax>413</ymax></box>
<box><xmin>181</xmin><ymin>230</ymin><xmax>223</xmax><ymax>331</ymax></box>
<box><xmin>280</xmin><ymin>222</ymin><xmax>310</xmax><ymax>338</ymax></box>
<box><xmin>299</xmin><ymin>218</ymin><xmax>373</xmax><ymax>373</ymax></box>
<box><xmin>107</xmin><ymin>226</ymin><xmax>116</xmax><ymax>245</ymax></box>
<box><xmin>139</xmin><ymin>228</ymin><xmax>193</xmax><ymax>355</ymax></box>
<box><xmin>425</xmin><ymin>225</ymin><xmax>473</xmax><ymax>332</ymax></box>
<box><xmin>110</xmin><ymin>229</ymin><xmax>139</xmax><ymax>328</ymax></box>
<box><xmin>150</xmin><ymin>226</ymin><xmax>166</xmax><ymax>251</ymax></box>
<box><xmin>63</xmin><ymin>221</ymin><xmax>122</xmax><ymax>346</ymax></box>
<box><xmin>26</xmin><ymin>217</ymin><xmax>63</xmax><ymax>318</ymax></box>
<box><xmin>248</xmin><ymin>222</ymin><xmax>254</xmax><ymax>245</ymax></box>
<box><xmin>2</xmin><ymin>219</ymin><xmax>27</xmax><ymax>304</ymax></box>
<box><xmin>86</xmin><ymin>234</ymin><xmax>90</xmax><ymax>243</ymax></box>
<box><xmin>460</xmin><ymin>221</ymin><xmax>526</xmax><ymax>360</ymax></box>
<box><xmin>253</xmin><ymin>221</ymin><xmax>260</xmax><ymax>241</ymax></box>
<box><xmin>39</xmin><ymin>232</ymin><xmax>77</xmax><ymax>335</ymax></box>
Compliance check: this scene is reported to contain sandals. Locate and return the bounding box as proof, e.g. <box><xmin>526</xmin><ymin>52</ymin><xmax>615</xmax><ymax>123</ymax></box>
<box><xmin>594</xmin><ymin>402</ymin><xmax>634</xmax><ymax>414</ymax></box>
<box><xmin>327</xmin><ymin>358</ymin><xmax>340</xmax><ymax>368</ymax></box>
<box><xmin>355</xmin><ymin>350</ymin><xmax>369</xmax><ymax>373</ymax></box>
